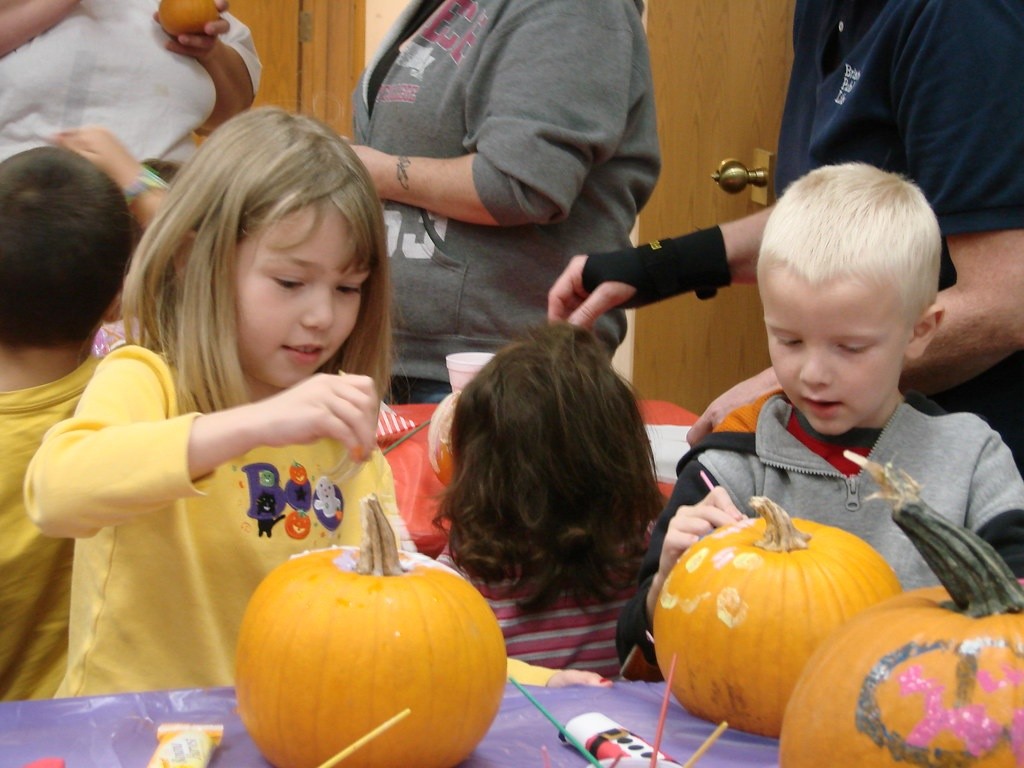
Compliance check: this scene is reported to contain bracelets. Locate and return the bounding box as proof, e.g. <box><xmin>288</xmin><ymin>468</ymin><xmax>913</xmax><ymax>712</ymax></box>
<box><xmin>123</xmin><ymin>165</ymin><xmax>170</xmax><ymax>204</ymax></box>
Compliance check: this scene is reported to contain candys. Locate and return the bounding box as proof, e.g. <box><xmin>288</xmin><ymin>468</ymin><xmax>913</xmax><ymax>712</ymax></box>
<box><xmin>143</xmin><ymin>722</ymin><xmax>223</xmax><ymax>768</ymax></box>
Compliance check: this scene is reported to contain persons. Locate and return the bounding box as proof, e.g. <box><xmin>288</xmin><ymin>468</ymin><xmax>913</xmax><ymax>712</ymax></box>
<box><xmin>0</xmin><ymin>0</ymin><xmax>262</xmax><ymax>168</ymax></box>
<box><xmin>23</xmin><ymin>108</ymin><xmax>419</xmax><ymax>699</ymax></box>
<box><xmin>616</xmin><ymin>161</ymin><xmax>1024</xmax><ymax>682</ymax></box>
<box><xmin>431</xmin><ymin>324</ymin><xmax>670</xmax><ymax>683</ymax></box>
<box><xmin>346</xmin><ymin>0</ymin><xmax>662</xmax><ymax>404</ymax></box>
<box><xmin>0</xmin><ymin>127</ymin><xmax>171</xmax><ymax>700</ymax></box>
<box><xmin>547</xmin><ymin>0</ymin><xmax>1024</xmax><ymax>476</ymax></box>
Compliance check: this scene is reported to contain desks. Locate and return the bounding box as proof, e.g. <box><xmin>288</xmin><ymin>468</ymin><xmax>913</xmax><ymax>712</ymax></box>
<box><xmin>378</xmin><ymin>398</ymin><xmax>702</xmax><ymax>562</ymax></box>
<box><xmin>0</xmin><ymin>679</ymin><xmax>781</xmax><ymax>768</ymax></box>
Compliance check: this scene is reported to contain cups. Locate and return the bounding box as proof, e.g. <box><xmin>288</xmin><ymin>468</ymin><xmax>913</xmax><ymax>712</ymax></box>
<box><xmin>446</xmin><ymin>351</ymin><xmax>496</xmax><ymax>391</ymax></box>
